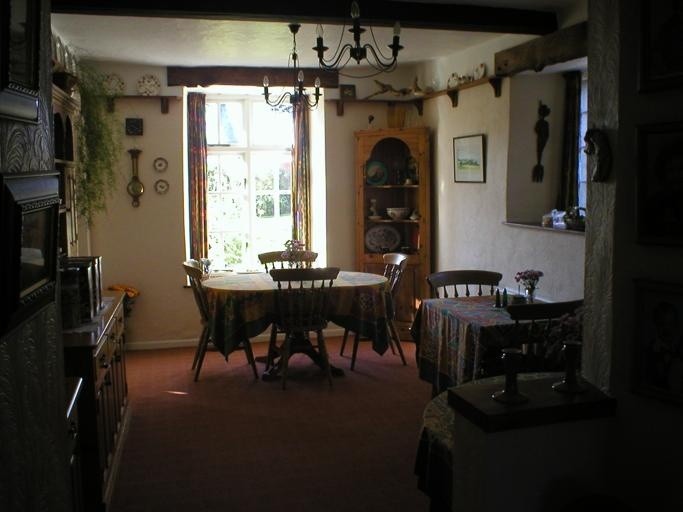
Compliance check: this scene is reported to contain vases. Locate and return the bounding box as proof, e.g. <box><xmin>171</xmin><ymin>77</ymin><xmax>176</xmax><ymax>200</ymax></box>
<box><xmin>525</xmin><ymin>287</ymin><xmax>538</xmax><ymax>305</ymax></box>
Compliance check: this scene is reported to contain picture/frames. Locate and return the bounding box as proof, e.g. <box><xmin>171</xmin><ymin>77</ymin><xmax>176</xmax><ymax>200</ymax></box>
<box><xmin>632</xmin><ymin>278</ymin><xmax>683</xmax><ymax>399</ymax></box>
<box><xmin>453</xmin><ymin>134</ymin><xmax>487</xmax><ymax>183</ymax></box>
<box><xmin>0</xmin><ymin>170</ymin><xmax>66</xmax><ymax>336</ymax></box>
<box><xmin>0</xmin><ymin>0</ymin><xmax>45</xmax><ymax>125</ymax></box>
<box><xmin>636</xmin><ymin>1</ymin><xmax>683</xmax><ymax>94</ymax></box>
<box><xmin>633</xmin><ymin>122</ymin><xmax>683</xmax><ymax>247</ymax></box>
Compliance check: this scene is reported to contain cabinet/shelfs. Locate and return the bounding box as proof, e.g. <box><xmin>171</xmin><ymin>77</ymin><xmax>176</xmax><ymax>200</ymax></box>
<box><xmin>68</xmin><ymin>293</ymin><xmax>132</xmax><ymax>512</ymax></box>
<box><xmin>353</xmin><ymin>128</ymin><xmax>433</xmax><ymax>322</ymax></box>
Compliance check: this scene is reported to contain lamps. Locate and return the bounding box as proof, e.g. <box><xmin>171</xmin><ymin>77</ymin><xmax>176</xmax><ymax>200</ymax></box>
<box><xmin>314</xmin><ymin>0</ymin><xmax>404</xmax><ymax>75</ymax></box>
<box><xmin>261</xmin><ymin>24</ymin><xmax>322</xmax><ymax>112</ymax></box>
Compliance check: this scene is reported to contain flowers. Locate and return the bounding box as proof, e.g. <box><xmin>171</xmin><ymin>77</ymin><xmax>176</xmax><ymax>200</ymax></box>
<box><xmin>515</xmin><ymin>270</ymin><xmax>544</xmax><ymax>295</ymax></box>
<box><xmin>105</xmin><ymin>284</ymin><xmax>140</xmax><ymax>324</ymax></box>
<box><xmin>281</xmin><ymin>240</ymin><xmax>312</xmax><ymax>268</ymax></box>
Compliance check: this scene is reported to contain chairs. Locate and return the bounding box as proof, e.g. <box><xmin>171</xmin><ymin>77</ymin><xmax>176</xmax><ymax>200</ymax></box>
<box><xmin>427</xmin><ymin>270</ymin><xmax>502</xmax><ymax>298</ymax></box>
<box><xmin>506</xmin><ymin>299</ymin><xmax>584</xmax><ymax>338</ymax></box>
<box><xmin>183</xmin><ymin>253</ymin><xmax>407</xmax><ymax>391</ymax></box>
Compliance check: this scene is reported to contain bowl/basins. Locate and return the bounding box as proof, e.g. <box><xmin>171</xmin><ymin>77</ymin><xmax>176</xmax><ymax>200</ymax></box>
<box><xmin>386</xmin><ymin>207</ymin><xmax>411</xmax><ymax>219</ymax></box>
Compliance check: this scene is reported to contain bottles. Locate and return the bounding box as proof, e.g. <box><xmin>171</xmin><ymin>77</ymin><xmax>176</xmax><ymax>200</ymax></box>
<box><xmin>496</xmin><ymin>288</ymin><xmax>501</xmax><ymax>307</ymax></box>
<box><xmin>503</xmin><ymin>288</ymin><xmax>507</xmax><ymax>306</ymax></box>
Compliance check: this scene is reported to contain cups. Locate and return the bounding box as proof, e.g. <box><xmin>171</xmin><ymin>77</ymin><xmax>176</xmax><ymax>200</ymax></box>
<box><xmin>458</xmin><ymin>74</ymin><xmax>472</xmax><ymax>84</ymax></box>
<box><xmin>541</xmin><ymin>216</ymin><xmax>553</xmax><ymax>227</ymax></box>
<box><xmin>401</xmin><ymin>247</ymin><xmax>411</xmax><ymax>253</ymax></box>
<box><xmin>405</xmin><ymin>178</ymin><xmax>412</xmax><ymax>185</ymax></box>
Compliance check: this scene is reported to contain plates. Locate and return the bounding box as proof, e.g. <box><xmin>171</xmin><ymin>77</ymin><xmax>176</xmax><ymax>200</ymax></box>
<box><xmin>365</xmin><ymin>161</ymin><xmax>387</xmax><ymax>186</ymax></box>
<box><xmin>367</xmin><ymin>215</ymin><xmax>381</xmax><ymax>219</ymax></box>
<box><xmin>447</xmin><ymin>72</ymin><xmax>458</xmax><ymax>89</ymax></box>
<box><xmin>153</xmin><ymin>157</ymin><xmax>168</xmax><ymax>172</ymax></box>
<box><xmin>365</xmin><ymin>226</ymin><xmax>400</xmax><ymax>252</ymax></box>
<box><xmin>474</xmin><ymin>63</ymin><xmax>485</xmax><ymax>80</ymax></box>
<box><xmin>154</xmin><ymin>179</ymin><xmax>169</xmax><ymax>195</ymax></box>
<box><xmin>102</xmin><ymin>73</ymin><xmax>127</xmax><ymax>96</ymax></box>
<box><xmin>51</xmin><ymin>35</ymin><xmax>82</xmax><ymax>76</ymax></box>
<box><xmin>137</xmin><ymin>73</ymin><xmax>162</xmax><ymax>96</ymax></box>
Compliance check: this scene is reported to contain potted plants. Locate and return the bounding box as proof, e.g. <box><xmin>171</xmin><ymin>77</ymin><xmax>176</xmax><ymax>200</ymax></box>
<box><xmin>53</xmin><ymin>63</ymin><xmax>126</xmax><ymax>227</ymax></box>
